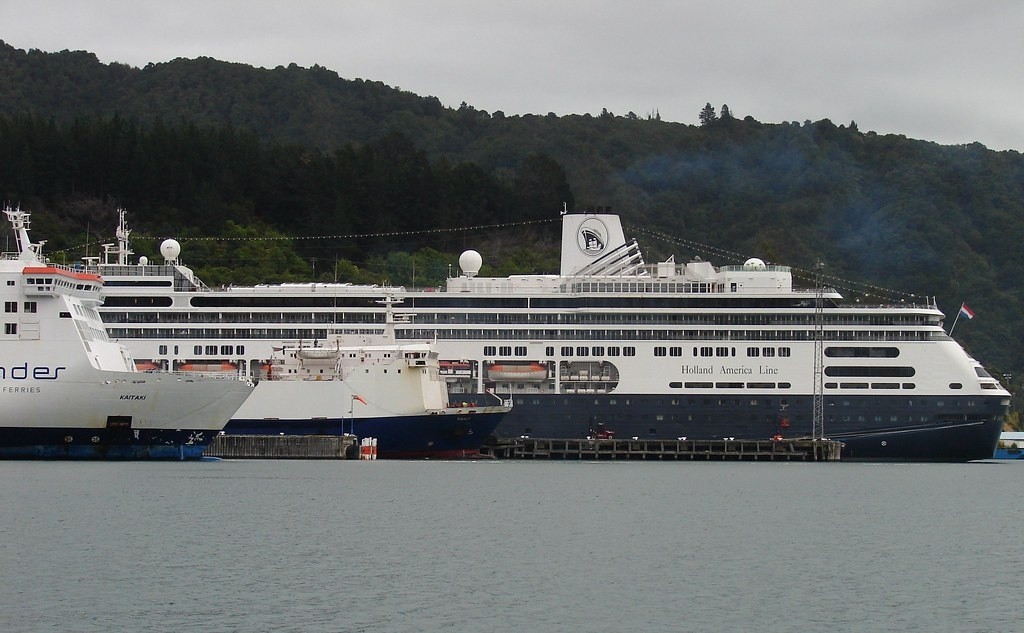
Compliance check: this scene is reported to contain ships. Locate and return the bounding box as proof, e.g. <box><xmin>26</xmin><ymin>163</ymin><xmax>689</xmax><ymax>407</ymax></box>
<box><xmin>72</xmin><ymin>202</ymin><xmax>1013</xmax><ymax>462</ymax></box>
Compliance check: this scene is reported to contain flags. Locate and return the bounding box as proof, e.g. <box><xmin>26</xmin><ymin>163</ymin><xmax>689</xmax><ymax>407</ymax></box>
<box><xmin>960</xmin><ymin>305</ymin><xmax>974</xmax><ymax>320</ymax></box>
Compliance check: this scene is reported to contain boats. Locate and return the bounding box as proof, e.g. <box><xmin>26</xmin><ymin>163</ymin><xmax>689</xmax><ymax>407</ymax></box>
<box><xmin>135</xmin><ymin>360</ymin><xmax>161</xmax><ymax>372</ymax></box>
<box><xmin>206</xmin><ymin>293</ymin><xmax>516</xmax><ymax>459</ymax></box>
<box><xmin>0</xmin><ymin>205</ymin><xmax>260</xmax><ymax>463</ymax></box>
<box><xmin>178</xmin><ymin>360</ymin><xmax>239</xmax><ymax>374</ymax></box>
<box><xmin>487</xmin><ymin>362</ymin><xmax>541</xmax><ymax>382</ymax></box>
